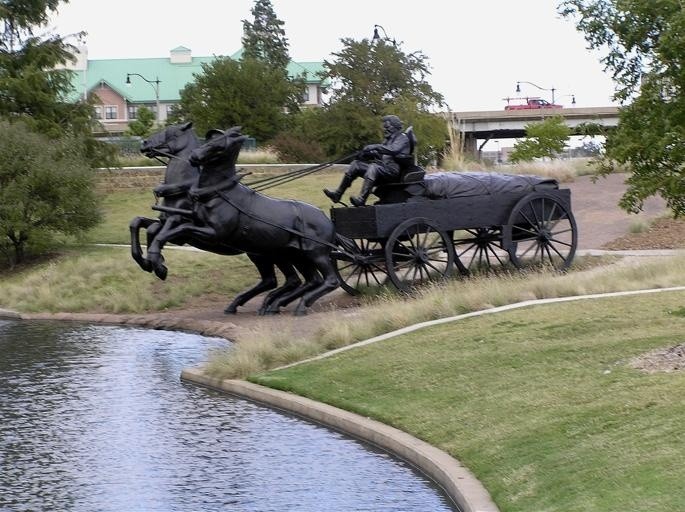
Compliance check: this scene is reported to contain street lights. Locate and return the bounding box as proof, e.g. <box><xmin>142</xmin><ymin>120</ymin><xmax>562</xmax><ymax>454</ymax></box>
<box><xmin>126</xmin><ymin>73</ymin><xmax>162</xmax><ymax>133</ymax></box>
<box><xmin>373</xmin><ymin>23</ymin><xmax>399</xmax><ymax>99</ymax></box>
<box><xmin>517</xmin><ymin>81</ymin><xmax>575</xmax><ymax>107</ymax></box>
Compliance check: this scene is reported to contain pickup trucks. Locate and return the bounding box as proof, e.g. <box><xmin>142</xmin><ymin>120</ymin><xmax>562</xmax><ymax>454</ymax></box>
<box><xmin>507</xmin><ymin>99</ymin><xmax>562</xmax><ymax>109</ymax></box>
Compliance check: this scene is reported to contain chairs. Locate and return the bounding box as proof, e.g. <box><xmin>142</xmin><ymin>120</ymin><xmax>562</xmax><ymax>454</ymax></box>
<box><xmin>372</xmin><ymin>128</ymin><xmax>425</xmax><ymax>202</ymax></box>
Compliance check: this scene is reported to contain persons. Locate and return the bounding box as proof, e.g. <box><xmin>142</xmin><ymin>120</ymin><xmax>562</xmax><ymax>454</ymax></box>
<box><xmin>322</xmin><ymin>114</ymin><xmax>419</xmax><ymax>207</ymax></box>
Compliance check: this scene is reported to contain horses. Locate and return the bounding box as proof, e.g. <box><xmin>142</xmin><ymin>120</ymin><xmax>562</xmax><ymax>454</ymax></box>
<box><xmin>127</xmin><ymin>118</ymin><xmax>360</xmax><ymax>316</ymax></box>
